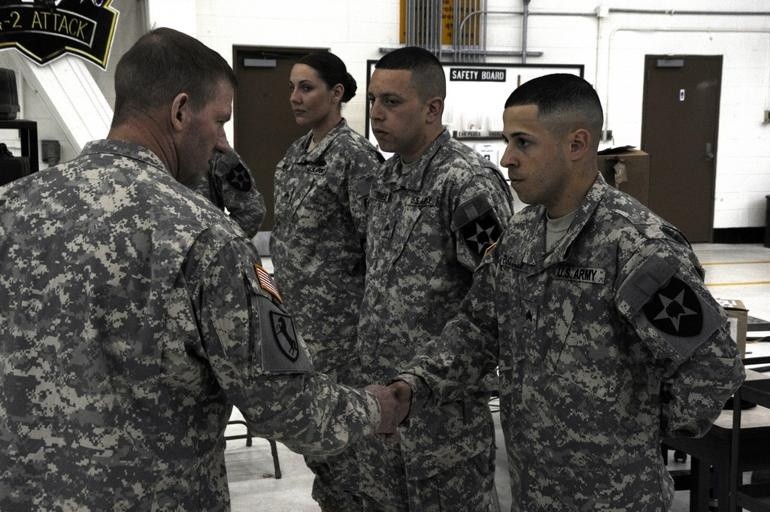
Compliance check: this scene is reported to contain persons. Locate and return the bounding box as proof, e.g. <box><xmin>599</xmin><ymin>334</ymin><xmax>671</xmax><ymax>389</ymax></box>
<box><xmin>381</xmin><ymin>69</ymin><xmax>749</xmax><ymax>511</ymax></box>
<box><xmin>0</xmin><ymin>24</ymin><xmax>402</xmax><ymax>509</ymax></box>
<box><xmin>266</xmin><ymin>49</ymin><xmax>387</xmax><ymax>509</ymax></box>
<box><xmin>197</xmin><ymin>138</ymin><xmax>269</xmax><ymax>245</ymax></box>
<box><xmin>342</xmin><ymin>42</ymin><xmax>517</xmax><ymax>512</ymax></box>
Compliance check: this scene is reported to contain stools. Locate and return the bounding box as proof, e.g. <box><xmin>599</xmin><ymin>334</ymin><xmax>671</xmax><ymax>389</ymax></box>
<box><xmin>225</xmin><ymin>404</ymin><xmax>283</xmax><ymax>479</ymax></box>
<box><xmin>660</xmin><ymin>315</ymin><xmax>770</xmax><ymax>512</ymax></box>
